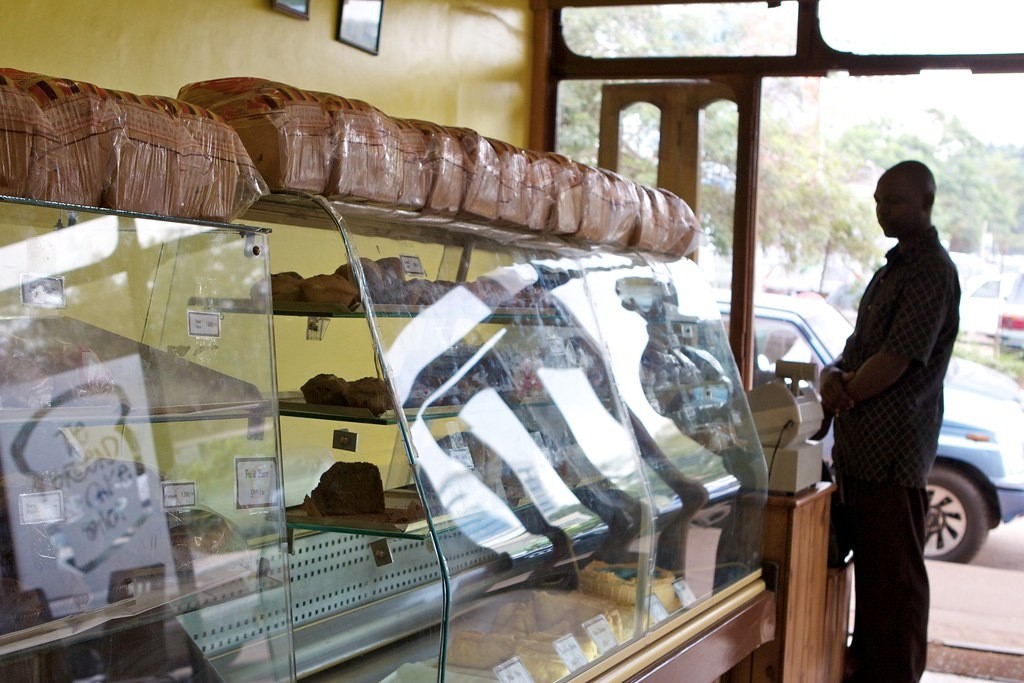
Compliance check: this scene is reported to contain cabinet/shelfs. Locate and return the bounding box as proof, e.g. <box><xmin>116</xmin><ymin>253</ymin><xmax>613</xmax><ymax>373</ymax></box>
<box><xmin>189</xmin><ymin>297</ymin><xmax>644</xmax><ymax>540</ymax></box>
<box><xmin>720</xmin><ymin>483</ymin><xmax>854</xmax><ymax>683</ymax></box>
<box><xmin>0</xmin><ymin>312</ymin><xmax>271</xmax><ymax>660</ymax></box>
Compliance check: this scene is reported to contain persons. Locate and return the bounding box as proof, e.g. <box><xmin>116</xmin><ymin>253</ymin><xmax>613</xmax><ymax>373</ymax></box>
<box><xmin>615</xmin><ymin>276</ymin><xmax>765</xmax><ymax>588</ymax></box>
<box><xmin>819</xmin><ymin>161</ymin><xmax>961</xmax><ymax>682</ymax></box>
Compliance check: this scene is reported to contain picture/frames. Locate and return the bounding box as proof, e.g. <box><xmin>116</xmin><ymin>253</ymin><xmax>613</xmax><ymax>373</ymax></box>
<box><xmin>336</xmin><ymin>0</ymin><xmax>384</xmax><ymax>55</ymax></box>
<box><xmin>271</xmin><ymin>0</ymin><xmax>310</xmax><ymax>20</ymax></box>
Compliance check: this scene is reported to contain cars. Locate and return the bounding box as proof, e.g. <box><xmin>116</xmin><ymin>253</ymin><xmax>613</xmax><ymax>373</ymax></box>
<box><xmin>960</xmin><ymin>277</ymin><xmax>1024</xmax><ymax>349</ymax></box>
<box><xmin>717</xmin><ymin>291</ymin><xmax>1024</xmax><ymax>566</ymax></box>
